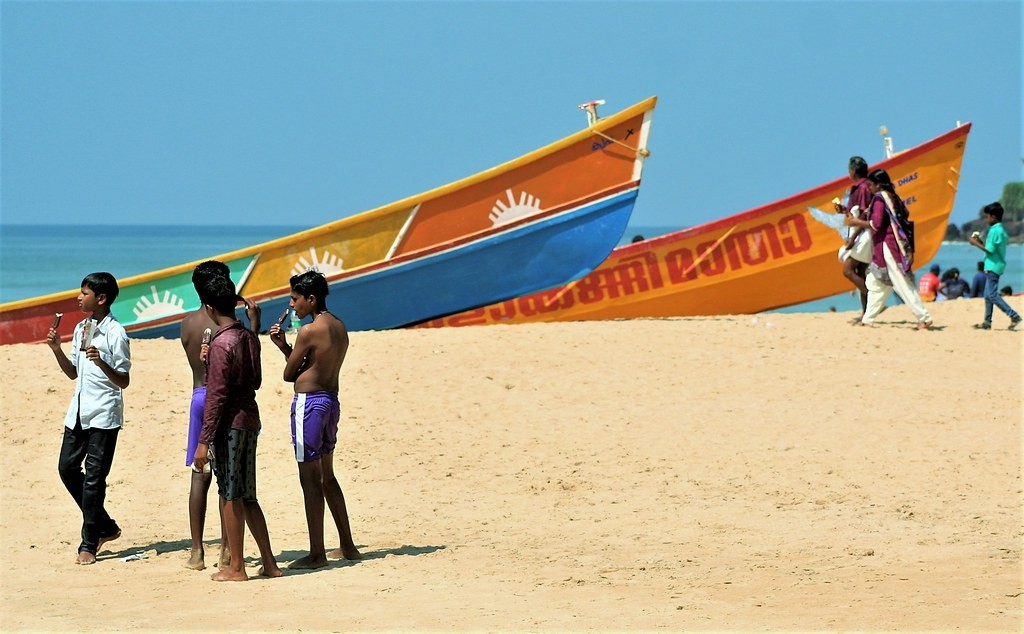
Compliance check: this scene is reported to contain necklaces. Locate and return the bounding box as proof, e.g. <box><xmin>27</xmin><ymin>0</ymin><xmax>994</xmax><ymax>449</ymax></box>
<box><xmin>313</xmin><ymin>310</ymin><xmax>330</xmax><ymax>322</ymax></box>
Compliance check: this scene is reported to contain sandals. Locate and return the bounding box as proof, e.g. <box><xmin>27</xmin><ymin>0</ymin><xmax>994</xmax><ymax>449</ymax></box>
<box><xmin>973</xmin><ymin>323</ymin><xmax>992</xmax><ymax>330</ymax></box>
<box><xmin>1008</xmin><ymin>316</ymin><xmax>1022</xmax><ymax>331</ymax></box>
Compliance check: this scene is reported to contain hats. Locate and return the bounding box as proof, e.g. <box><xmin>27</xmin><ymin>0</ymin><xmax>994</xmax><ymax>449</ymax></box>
<box><xmin>931</xmin><ymin>264</ymin><xmax>942</xmax><ymax>272</ymax></box>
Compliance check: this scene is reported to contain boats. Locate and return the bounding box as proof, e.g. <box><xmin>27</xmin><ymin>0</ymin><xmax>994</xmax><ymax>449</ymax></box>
<box><xmin>397</xmin><ymin>121</ymin><xmax>973</xmax><ymax>331</ymax></box>
<box><xmin>1</xmin><ymin>94</ymin><xmax>662</xmax><ymax>346</ymax></box>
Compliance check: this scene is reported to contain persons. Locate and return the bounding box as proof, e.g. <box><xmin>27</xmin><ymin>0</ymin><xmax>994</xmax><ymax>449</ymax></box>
<box><xmin>968</xmin><ymin>203</ymin><xmax>1022</xmax><ymax>331</ymax></box>
<box><xmin>834</xmin><ymin>156</ymin><xmax>886</xmax><ymax>325</ymax></box>
<box><xmin>270</xmin><ymin>271</ymin><xmax>363</xmax><ymax>568</ymax></box>
<box><xmin>848</xmin><ymin>169</ymin><xmax>933</xmax><ymax>331</ymax></box>
<box><xmin>46</xmin><ymin>272</ymin><xmax>132</xmax><ymax>564</ymax></box>
<box><xmin>918</xmin><ymin>261</ymin><xmax>1012</xmax><ymax>302</ymax></box>
<box><xmin>194</xmin><ymin>276</ymin><xmax>283</xmax><ymax>581</ymax></box>
<box><xmin>179</xmin><ymin>261</ymin><xmax>261</xmax><ymax>571</ymax></box>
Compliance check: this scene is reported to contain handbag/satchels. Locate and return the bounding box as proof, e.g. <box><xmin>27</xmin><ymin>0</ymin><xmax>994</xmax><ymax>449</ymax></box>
<box><xmin>838</xmin><ymin>245</ymin><xmax>852</xmax><ymax>262</ymax></box>
